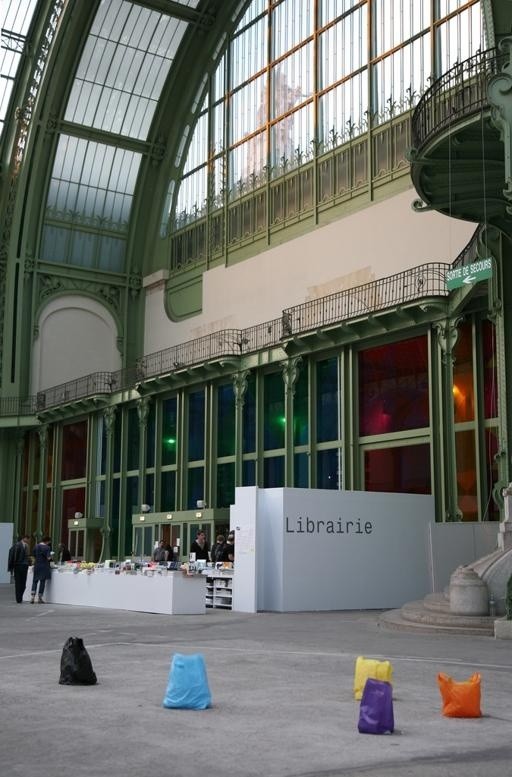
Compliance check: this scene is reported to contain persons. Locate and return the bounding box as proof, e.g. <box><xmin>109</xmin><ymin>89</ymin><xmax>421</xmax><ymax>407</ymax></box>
<box><xmin>7</xmin><ymin>534</ymin><xmax>71</xmax><ymax>604</ymax></box>
<box><xmin>152</xmin><ymin>528</ymin><xmax>235</xmax><ymax>573</ymax></box>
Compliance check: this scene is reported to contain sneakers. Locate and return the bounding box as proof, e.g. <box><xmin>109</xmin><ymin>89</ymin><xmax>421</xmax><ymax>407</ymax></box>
<box><xmin>29</xmin><ymin>598</ymin><xmax>45</xmax><ymax>604</ymax></box>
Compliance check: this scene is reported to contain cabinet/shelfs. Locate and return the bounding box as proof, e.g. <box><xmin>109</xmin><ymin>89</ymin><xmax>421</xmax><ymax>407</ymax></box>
<box><xmin>202</xmin><ymin>574</ymin><xmax>232</xmax><ymax>608</ymax></box>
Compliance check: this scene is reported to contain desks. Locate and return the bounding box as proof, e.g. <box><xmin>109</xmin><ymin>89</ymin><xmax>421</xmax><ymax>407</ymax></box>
<box><xmin>23</xmin><ymin>567</ymin><xmax>206</xmax><ymax>615</ymax></box>
